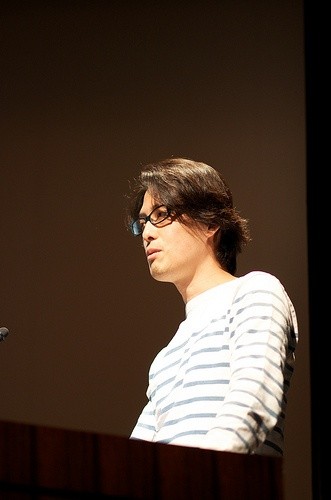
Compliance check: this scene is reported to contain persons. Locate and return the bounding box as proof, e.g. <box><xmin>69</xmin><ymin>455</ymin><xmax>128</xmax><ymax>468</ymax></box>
<box><xmin>122</xmin><ymin>156</ymin><xmax>298</xmax><ymax>456</ymax></box>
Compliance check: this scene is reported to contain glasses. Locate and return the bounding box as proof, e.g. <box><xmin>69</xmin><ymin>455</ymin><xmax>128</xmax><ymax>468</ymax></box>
<box><xmin>132</xmin><ymin>204</ymin><xmax>195</xmax><ymax>236</ymax></box>
